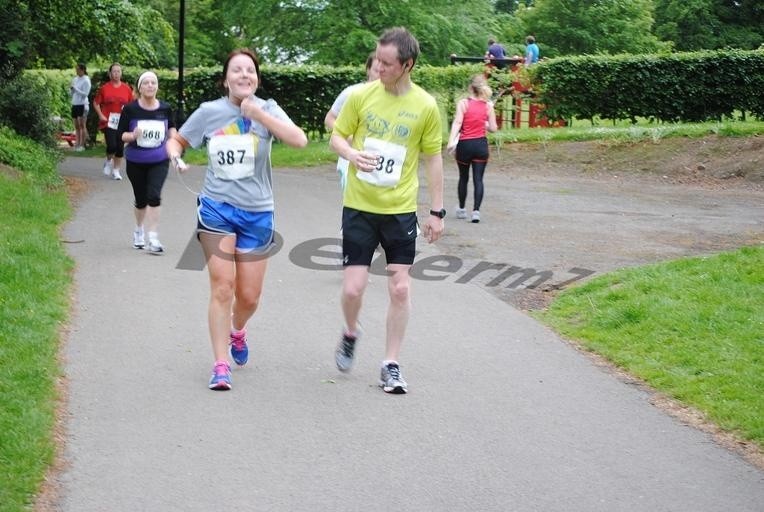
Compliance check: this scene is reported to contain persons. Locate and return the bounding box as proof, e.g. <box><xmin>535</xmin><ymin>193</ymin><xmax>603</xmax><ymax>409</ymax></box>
<box><xmin>70</xmin><ymin>64</ymin><xmax>91</xmax><ymax>152</ymax></box>
<box><xmin>488</xmin><ymin>39</ymin><xmax>506</xmax><ymax>69</ymax></box>
<box><xmin>329</xmin><ymin>26</ymin><xmax>447</xmax><ymax>394</ymax></box>
<box><xmin>93</xmin><ymin>64</ymin><xmax>137</xmax><ymax>180</ymax></box>
<box><xmin>325</xmin><ymin>50</ymin><xmax>383</xmax><ymax>247</ymax></box>
<box><xmin>447</xmin><ymin>76</ymin><xmax>497</xmax><ymax>222</ymax></box>
<box><xmin>117</xmin><ymin>71</ymin><xmax>178</xmax><ymax>252</ymax></box>
<box><xmin>526</xmin><ymin>36</ymin><xmax>540</xmax><ymax>67</ymax></box>
<box><xmin>165</xmin><ymin>48</ymin><xmax>307</xmax><ymax>390</ymax></box>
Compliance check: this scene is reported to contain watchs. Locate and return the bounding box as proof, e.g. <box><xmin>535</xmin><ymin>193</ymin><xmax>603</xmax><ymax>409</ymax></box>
<box><xmin>430</xmin><ymin>208</ymin><xmax>447</xmax><ymax>218</ymax></box>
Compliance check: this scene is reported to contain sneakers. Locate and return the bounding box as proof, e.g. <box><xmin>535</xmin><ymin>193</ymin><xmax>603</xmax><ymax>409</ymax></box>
<box><xmin>471</xmin><ymin>209</ymin><xmax>481</xmax><ymax>223</ymax></box>
<box><xmin>148</xmin><ymin>239</ymin><xmax>164</xmax><ymax>252</ymax></box>
<box><xmin>113</xmin><ymin>168</ymin><xmax>122</xmax><ymax>180</ymax></box>
<box><xmin>134</xmin><ymin>230</ymin><xmax>145</xmax><ymax>247</ymax></box>
<box><xmin>456</xmin><ymin>206</ymin><xmax>467</xmax><ymax>219</ymax></box>
<box><xmin>103</xmin><ymin>159</ymin><xmax>111</xmax><ymax>175</ymax></box>
<box><xmin>378</xmin><ymin>361</ymin><xmax>409</xmax><ymax>394</ymax></box>
<box><xmin>336</xmin><ymin>331</ymin><xmax>357</xmax><ymax>371</ymax></box>
<box><xmin>208</xmin><ymin>360</ymin><xmax>232</xmax><ymax>392</ymax></box>
<box><xmin>228</xmin><ymin>329</ymin><xmax>249</xmax><ymax>367</ymax></box>
